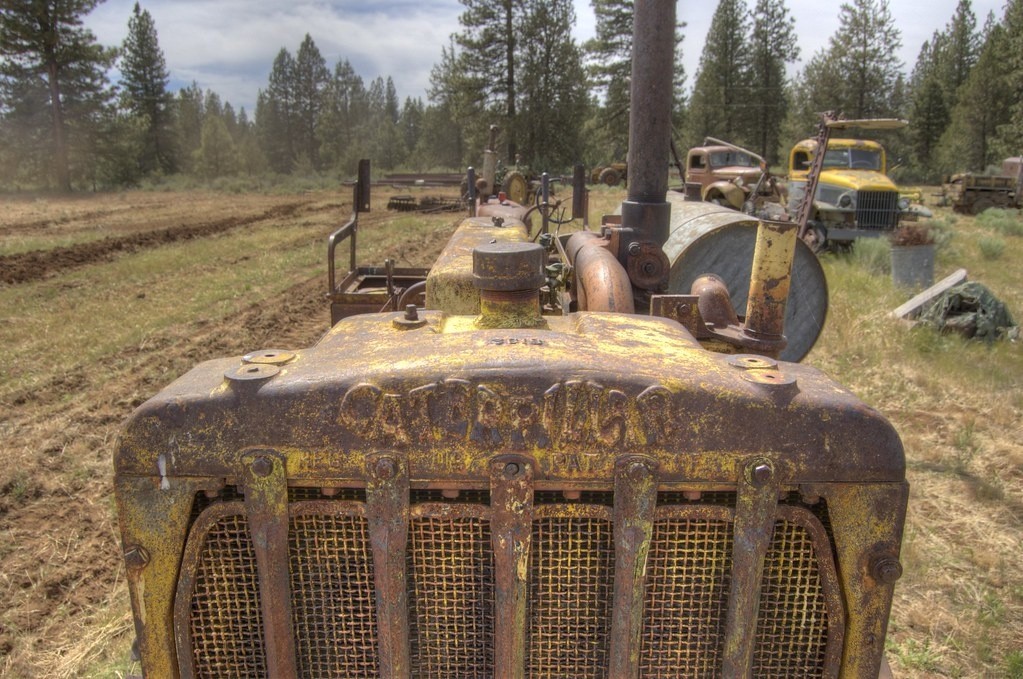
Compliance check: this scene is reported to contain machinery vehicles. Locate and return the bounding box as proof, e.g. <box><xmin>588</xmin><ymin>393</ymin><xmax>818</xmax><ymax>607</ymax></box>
<box><xmin>115</xmin><ymin>0</ymin><xmax>1023</xmax><ymax>679</ymax></box>
